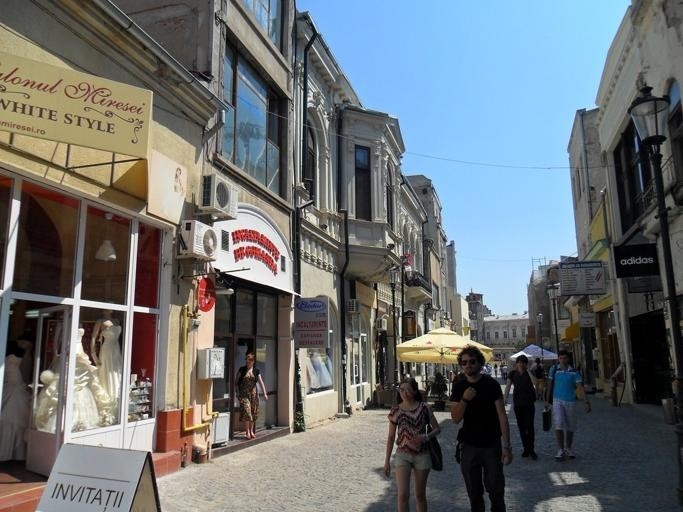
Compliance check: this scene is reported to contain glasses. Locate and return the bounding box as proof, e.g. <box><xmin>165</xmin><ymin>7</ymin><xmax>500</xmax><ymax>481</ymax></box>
<box><xmin>398</xmin><ymin>386</ymin><xmax>412</xmax><ymax>393</ymax></box>
<box><xmin>460</xmin><ymin>358</ymin><xmax>478</xmax><ymax>366</ymax></box>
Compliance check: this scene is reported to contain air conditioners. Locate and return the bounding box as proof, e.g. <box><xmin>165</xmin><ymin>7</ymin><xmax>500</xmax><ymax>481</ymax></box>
<box><xmin>375</xmin><ymin>318</ymin><xmax>389</xmax><ymax>332</ymax></box>
<box><xmin>175</xmin><ymin>169</ymin><xmax>240</xmax><ymax>259</ymax></box>
<box><xmin>347</xmin><ymin>298</ymin><xmax>360</xmax><ymax>313</ymax></box>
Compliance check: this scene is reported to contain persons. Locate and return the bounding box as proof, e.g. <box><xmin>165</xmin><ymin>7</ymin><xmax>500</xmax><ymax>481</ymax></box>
<box><xmin>234</xmin><ymin>352</ymin><xmax>269</xmax><ymax>441</ymax></box>
<box><xmin>449</xmin><ymin>346</ymin><xmax>514</xmax><ymax>512</ymax></box>
<box><xmin>486</xmin><ymin>346</ymin><xmax>575</xmax><ymax>401</ymax></box>
<box><xmin>383</xmin><ymin>376</ymin><xmax>442</xmax><ymax>512</ymax></box>
<box><xmin>543</xmin><ymin>348</ymin><xmax>593</xmax><ymax>462</ymax></box>
<box><xmin>502</xmin><ymin>354</ymin><xmax>540</xmax><ymax>461</ymax></box>
<box><xmin>1</xmin><ymin>295</ymin><xmax>122</xmax><ymax>466</ymax></box>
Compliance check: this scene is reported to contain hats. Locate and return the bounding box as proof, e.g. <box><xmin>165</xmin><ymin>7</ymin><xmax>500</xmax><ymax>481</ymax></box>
<box><xmin>534</xmin><ymin>358</ymin><xmax>541</xmax><ymax>362</ymax></box>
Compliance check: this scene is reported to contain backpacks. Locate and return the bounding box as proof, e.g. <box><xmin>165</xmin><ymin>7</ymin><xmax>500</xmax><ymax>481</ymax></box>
<box><xmin>535</xmin><ymin>365</ymin><xmax>543</xmax><ymax>379</ymax></box>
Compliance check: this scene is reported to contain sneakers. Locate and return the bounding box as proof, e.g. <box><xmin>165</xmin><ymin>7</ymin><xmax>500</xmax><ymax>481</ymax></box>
<box><xmin>521</xmin><ymin>446</ymin><xmax>575</xmax><ymax>461</ymax></box>
<box><xmin>246</xmin><ymin>432</ymin><xmax>255</xmax><ymax>440</ymax></box>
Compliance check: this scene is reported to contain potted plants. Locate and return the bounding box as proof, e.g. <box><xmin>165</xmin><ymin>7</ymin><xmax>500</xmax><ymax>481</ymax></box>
<box><xmin>430</xmin><ymin>372</ymin><xmax>448</xmax><ymax>411</ymax></box>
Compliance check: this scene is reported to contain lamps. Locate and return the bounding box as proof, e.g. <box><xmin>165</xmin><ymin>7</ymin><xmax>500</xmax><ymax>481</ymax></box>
<box><xmin>94</xmin><ymin>213</ymin><xmax>117</xmax><ymax>263</ymax></box>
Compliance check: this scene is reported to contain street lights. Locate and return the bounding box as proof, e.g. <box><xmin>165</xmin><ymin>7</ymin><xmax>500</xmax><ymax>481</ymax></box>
<box><xmin>536</xmin><ymin>313</ymin><xmax>544</xmax><ymax>358</ymax></box>
<box><xmin>388</xmin><ymin>270</ymin><xmax>399</xmax><ymax>406</ymax></box>
<box><xmin>545</xmin><ymin>279</ymin><xmax>560</xmax><ymax>358</ymax></box>
<box><xmin>627</xmin><ymin>81</ymin><xmax>682</xmax><ymax>415</ymax></box>
<box><xmin>439</xmin><ymin>312</ymin><xmax>451</xmax><ymax>382</ymax></box>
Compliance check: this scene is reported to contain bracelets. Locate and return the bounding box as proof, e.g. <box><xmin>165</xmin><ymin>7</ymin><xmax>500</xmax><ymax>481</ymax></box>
<box><xmin>585</xmin><ymin>399</ymin><xmax>589</xmax><ymax>404</ymax></box>
<box><xmin>503</xmin><ymin>446</ymin><xmax>512</xmax><ymax>451</ymax></box>
<box><xmin>423</xmin><ymin>434</ymin><xmax>429</xmax><ymax>441</ymax></box>
<box><xmin>462</xmin><ymin>396</ymin><xmax>470</xmax><ymax>403</ymax></box>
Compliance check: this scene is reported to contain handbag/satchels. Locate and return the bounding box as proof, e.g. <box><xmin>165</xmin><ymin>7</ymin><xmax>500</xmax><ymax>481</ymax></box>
<box><xmin>424</xmin><ymin>407</ymin><xmax>443</xmax><ymax>471</ymax></box>
<box><xmin>541</xmin><ymin>406</ymin><xmax>552</xmax><ymax>432</ymax></box>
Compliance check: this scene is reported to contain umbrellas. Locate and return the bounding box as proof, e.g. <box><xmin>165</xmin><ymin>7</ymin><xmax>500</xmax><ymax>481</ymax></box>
<box><xmin>510</xmin><ymin>343</ymin><xmax>558</xmax><ymax>361</ymax></box>
<box><xmin>394</xmin><ymin>326</ymin><xmax>493</xmax><ymax>376</ymax></box>
